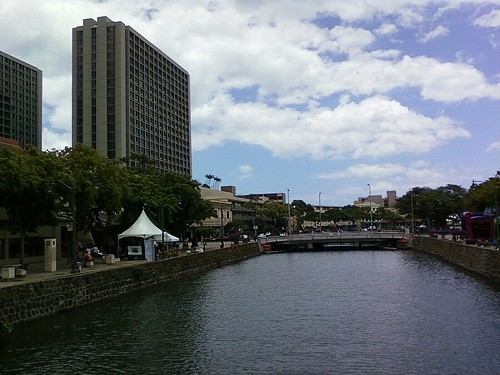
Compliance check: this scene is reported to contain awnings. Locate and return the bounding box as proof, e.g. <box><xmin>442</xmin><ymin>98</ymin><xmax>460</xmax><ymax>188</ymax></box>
<box><xmin>151</xmin><ymin>231</ymin><xmax>181</xmax><ymax>243</ymax></box>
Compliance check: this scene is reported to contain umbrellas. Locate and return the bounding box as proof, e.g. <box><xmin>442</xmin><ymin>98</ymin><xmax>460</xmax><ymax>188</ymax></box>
<box><xmin>118</xmin><ymin>211</ymin><xmax>168</xmax><ymax>240</ymax></box>
